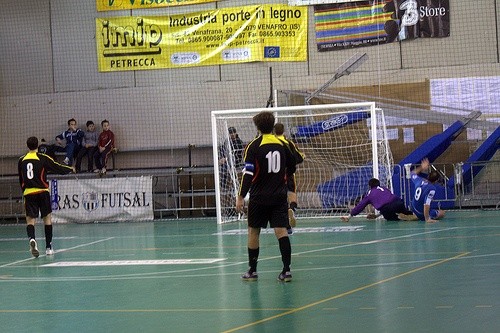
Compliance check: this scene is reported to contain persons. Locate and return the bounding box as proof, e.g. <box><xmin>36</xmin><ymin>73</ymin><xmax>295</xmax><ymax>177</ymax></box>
<box><xmin>55</xmin><ymin>118</ymin><xmax>82</xmax><ymax>166</ymax></box>
<box><xmin>93</xmin><ymin>119</ymin><xmax>115</xmax><ymax>174</ymax></box>
<box><xmin>75</xmin><ymin>120</ymin><xmax>99</xmax><ymax>174</ymax></box>
<box><xmin>275</xmin><ymin>122</ymin><xmax>305</xmax><ymax>227</ymax></box>
<box><xmin>236</xmin><ymin>111</ymin><xmax>297</xmax><ymax>282</ymax></box>
<box><xmin>17</xmin><ymin>136</ymin><xmax>76</xmax><ymax>258</ymax></box>
<box><xmin>409</xmin><ymin>157</ymin><xmax>445</xmax><ymax>222</ymax></box>
<box><xmin>218</xmin><ymin>126</ymin><xmax>243</xmax><ymax>219</ymax></box>
<box><xmin>340</xmin><ymin>178</ymin><xmax>413</xmax><ymax>222</ymax></box>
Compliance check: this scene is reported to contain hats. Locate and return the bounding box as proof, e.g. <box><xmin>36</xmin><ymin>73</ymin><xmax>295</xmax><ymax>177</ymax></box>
<box><xmin>228</xmin><ymin>127</ymin><xmax>236</xmax><ymax>132</ymax></box>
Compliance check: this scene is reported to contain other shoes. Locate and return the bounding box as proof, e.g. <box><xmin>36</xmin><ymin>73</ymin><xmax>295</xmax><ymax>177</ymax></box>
<box><xmin>101</xmin><ymin>167</ymin><xmax>106</xmax><ymax>173</ymax></box>
<box><xmin>64</xmin><ymin>157</ymin><xmax>71</xmax><ymax>165</ymax></box>
<box><xmin>29</xmin><ymin>239</ymin><xmax>39</xmax><ymax>258</ymax></box>
<box><xmin>288</xmin><ymin>209</ymin><xmax>296</xmax><ymax>228</ymax></box>
<box><xmin>46</xmin><ymin>248</ymin><xmax>53</xmax><ymax>255</ymax></box>
<box><xmin>93</xmin><ymin>169</ymin><xmax>101</xmax><ymax>173</ymax></box>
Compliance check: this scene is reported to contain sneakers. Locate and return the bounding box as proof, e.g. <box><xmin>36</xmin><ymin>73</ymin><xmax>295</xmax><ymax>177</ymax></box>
<box><xmin>277</xmin><ymin>271</ymin><xmax>292</xmax><ymax>282</ymax></box>
<box><xmin>240</xmin><ymin>272</ymin><xmax>258</xmax><ymax>281</ymax></box>
